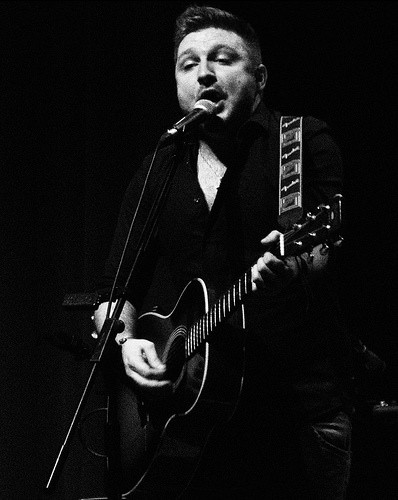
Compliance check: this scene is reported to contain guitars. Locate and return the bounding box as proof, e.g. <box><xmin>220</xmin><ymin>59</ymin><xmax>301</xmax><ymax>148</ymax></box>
<box><xmin>107</xmin><ymin>192</ymin><xmax>344</xmax><ymax>496</ymax></box>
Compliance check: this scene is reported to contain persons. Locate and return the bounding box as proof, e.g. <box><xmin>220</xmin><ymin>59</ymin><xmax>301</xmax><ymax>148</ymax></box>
<box><xmin>69</xmin><ymin>2</ymin><xmax>360</xmax><ymax>500</ymax></box>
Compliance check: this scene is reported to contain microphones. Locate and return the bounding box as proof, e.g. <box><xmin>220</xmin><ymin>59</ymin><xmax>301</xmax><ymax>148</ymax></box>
<box><xmin>159</xmin><ymin>99</ymin><xmax>218</xmax><ymax>143</ymax></box>
